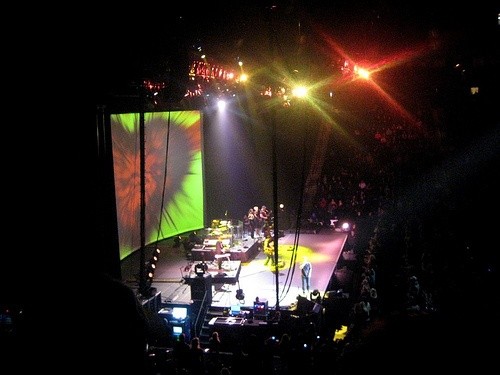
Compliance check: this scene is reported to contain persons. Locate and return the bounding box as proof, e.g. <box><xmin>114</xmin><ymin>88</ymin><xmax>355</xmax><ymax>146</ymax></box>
<box><xmin>253</xmin><ymin>206</ymin><xmax>262</xmax><ymax>236</ymax></box>
<box><xmin>216</xmin><ymin>237</ymin><xmax>225</xmax><ymax>269</ymax></box>
<box><xmin>262</xmin><ymin>221</ymin><xmax>271</xmax><ymax>238</ymax></box>
<box><xmin>260</xmin><ymin>205</ymin><xmax>273</xmax><ymax>236</ymax></box>
<box><xmin>178</xmin><ymin>293</ymin><xmax>323</xmax><ymax>371</ymax></box>
<box><xmin>300</xmin><ymin>256</ymin><xmax>314</xmax><ymax>294</ymax></box>
<box><xmin>305</xmin><ymin>117</ymin><xmax>426</xmax><ymax>318</ymax></box>
<box><xmin>263</xmin><ymin>233</ymin><xmax>274</xmax><ymax>265</ymax></box>
<box><xmin>249</xmin><ymin>208</ymin><xmax>257</xmax><ymax>238</ymax></box>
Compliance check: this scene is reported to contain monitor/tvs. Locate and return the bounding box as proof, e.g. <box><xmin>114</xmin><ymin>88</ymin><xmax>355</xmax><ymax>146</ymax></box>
<box><xmin>254</xmin><ymin>300</ymin><xmax>268</xmax><ymax>315</ymax></box>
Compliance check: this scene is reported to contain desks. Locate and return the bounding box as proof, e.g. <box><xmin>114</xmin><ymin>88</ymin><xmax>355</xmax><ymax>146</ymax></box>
<box><xmin>215</xmin><ymin>254</ymin><xmax>231</xmax><ymax>270</ymax></box>
